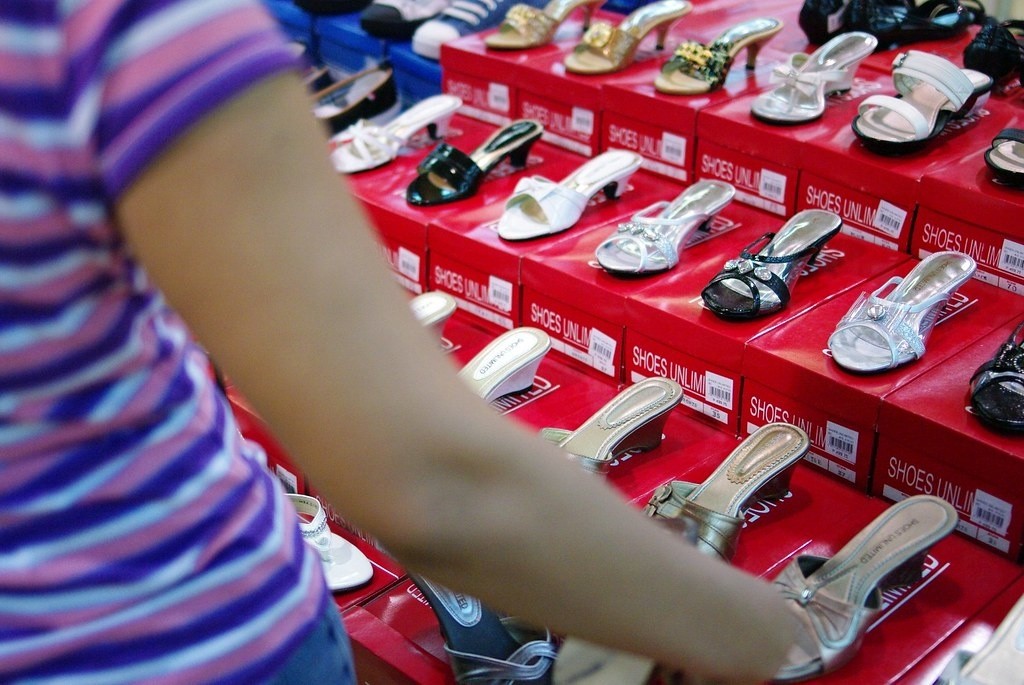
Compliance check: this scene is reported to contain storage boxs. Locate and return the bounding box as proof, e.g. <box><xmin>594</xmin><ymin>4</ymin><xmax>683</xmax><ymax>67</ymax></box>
<box><xmin>205</xmin><ymin>0</ymin><xmax>1024</xmax><ymax>685</ymax></box>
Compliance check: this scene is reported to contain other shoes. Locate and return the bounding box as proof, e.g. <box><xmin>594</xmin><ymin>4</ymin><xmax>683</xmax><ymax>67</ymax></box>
<box><xmin>354</xmin><ymin>0</ymin><xmax>451</xmax><ymax>42</ymax></box>
<box><xmin>300</xmin><ymin>61</ymin><xmax>402</xmax><ymax>144</ymax></box>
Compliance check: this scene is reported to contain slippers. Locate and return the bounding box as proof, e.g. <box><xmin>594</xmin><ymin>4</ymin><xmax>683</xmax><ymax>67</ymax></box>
<box><xmin>851</xmin><ymin>51</ymin><xmax>994</xmax><ymax>158</ymax></box>
<box><xmin>969</xmin><ymin>320</ymin><xmax>1024</xmax><ymax>437</ymax></box>
<box><xmin>983</xmin><ymin>128</ymin><xmax>1024</xmax><ymax>190</ymax></box>
<box><xmin>940</xmin><ymin>593</ymin><xmax>1023</xmax><ymax>685</ymax></box>
<box><xmin>799</xmin><ymin>0</ymin><xmax>1023</xmax><ymax>87</ymax></box>
<box><xmin>281</xmin><ymin>494</ymin><xmax>374</xmax><ymax>594</ymax></box>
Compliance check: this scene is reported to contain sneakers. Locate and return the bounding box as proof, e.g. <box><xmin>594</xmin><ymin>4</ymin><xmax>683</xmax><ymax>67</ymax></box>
<box><xmin>411</xmin><ymin>0</ymin><xmax>512</xmax><ymax>59</ymax></box>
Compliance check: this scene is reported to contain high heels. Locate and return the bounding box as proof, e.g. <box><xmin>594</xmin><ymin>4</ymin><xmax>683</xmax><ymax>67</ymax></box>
<box><xmin>457</xmin><ymin>327</ymin><xmax>552</xmax><ymax>403</ymax></box>
<box><xmin>539</xmin><ymin>377</ymin><xmax>683</xmax><ymax>478</ymax></box>
<box><xmin>639</xmin><ymin>423</ymin><xmax>810</xmax><ymax>566</ymax></box>
<box><xmin>700</xmin><ymin>209</ymin><xmax>842</xmax><ymax>322</ymax></box>
<box><xmin>595</xmin><ymin>180</ymin><xmax>735</xmax><ymax>280</ymax></box>
<box><xmin>827</xmin><ymin>251</ymin><xmax>977</xmax><ymax>376</ymax></box>
<box><xmin>767</xmin><ymin>495</ymin><xmax>958</xmax><ymax>683</ymax></box>
<box><xmin>329</xmin><ymin>95</ymin><xmax>642</xmax><ymax>245</ymax></box>
<box><xmin>484</xmin><ymin>0</ymin><xmax>879</xmax><ymax>124</ymax></box>
<box><xmin>403</xmin><ymin>560</ymin><xmax>558</xmax><ymax>685</ymax></box>
<box><xmin>408</xmin><ymin>292</ymin><xmax>457</xmax><ymax>350</ymax></box>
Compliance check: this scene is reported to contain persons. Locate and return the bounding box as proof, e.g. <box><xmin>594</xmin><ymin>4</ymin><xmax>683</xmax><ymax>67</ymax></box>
<box><xmin>0</xmin><ymin>0</ymin><xmax>804</xmax><ymax>685</ymax></box>
